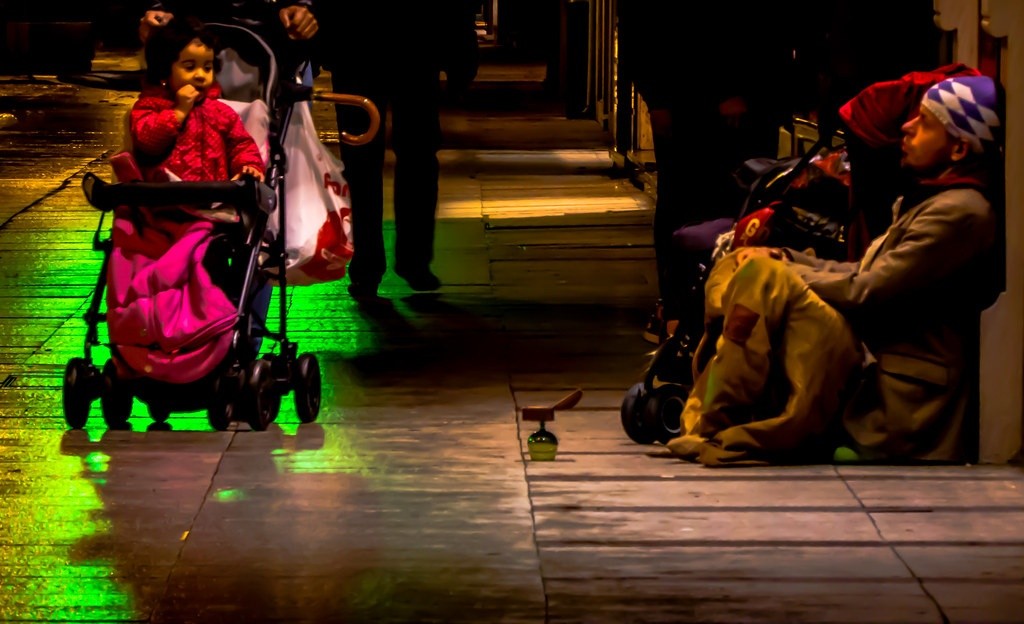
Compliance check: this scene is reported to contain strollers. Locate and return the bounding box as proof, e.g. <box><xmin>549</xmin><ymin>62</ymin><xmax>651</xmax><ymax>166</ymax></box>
<box><xmin>60</xmin><ymin>0</ymin><xmax>322</xmax><ymax>441</ymax></box>
<box><xmin>619</xmin><ymin>59</ymin><xmax>982</xmax><ymax>446</ymax></box>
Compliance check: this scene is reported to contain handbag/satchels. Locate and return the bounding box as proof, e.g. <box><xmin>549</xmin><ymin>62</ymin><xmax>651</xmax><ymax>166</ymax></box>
<box><xmin>255</xmin><ymin>69</ymin><xmax>356</xmax><ymax>286</ymax></box>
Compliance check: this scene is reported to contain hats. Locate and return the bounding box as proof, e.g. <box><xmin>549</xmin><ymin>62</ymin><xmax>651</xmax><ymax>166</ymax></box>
<box><xmin>921</xmin><ymin>76</ymin><xmax>1005</xmax><ymax>154</ymax></box>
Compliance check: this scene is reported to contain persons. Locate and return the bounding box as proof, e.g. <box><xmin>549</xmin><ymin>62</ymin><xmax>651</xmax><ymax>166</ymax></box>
<box><xmin>139</xmin><ymin>0</ymin><xmax>318</xmax><ymax>82</ymax></box>
<box><xmin>130</xmin><ymin>22</ymin><xmax>267</xmax><ymax>208</ymax></box>
<box><xmin>614</xmin><ymin>0</ymin><xmax>939</xmax><ymax>344</ymax></box>
<box><xmin>309</xmin><ymin>1</ymin><xmax>479</xmax><ymax>302</ymax></box>
<box><xmin>668</xmin><ymin>76</ymin><xmax>1006</xmax><ymax>467</ymax></box>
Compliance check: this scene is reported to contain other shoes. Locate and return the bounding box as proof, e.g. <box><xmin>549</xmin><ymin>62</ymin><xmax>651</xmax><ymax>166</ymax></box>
<box><xmin>642</xmin><ymin>300</ymin><xmax>665</xmax><ymax>344</ymax></box>
<box><xmin>394</xmin><ymin>262</ymin><xmax>441</xmax><ymax>291</ymax></box>
<box><xmin>346</xmin><ymin>276</ymin><xmax>376</xmax><ymax>307</ymax></box>
